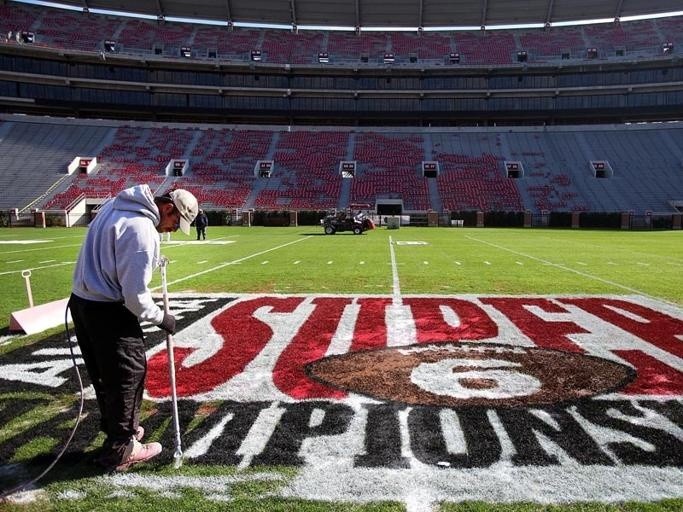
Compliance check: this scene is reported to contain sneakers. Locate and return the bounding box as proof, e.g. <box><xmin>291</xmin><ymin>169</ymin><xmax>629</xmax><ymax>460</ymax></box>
<box><xmin>103</xmin><ymin>424</ymin><xmax>145</xmax><ymax>450</ymax></box>
<box><xmin>110</xmin><ymin>441</ymin><xmax>164</xmax><ymax>471</ymax></box>
<box><xmin>196</xmin><ymin>237</ymin><xmax>206</xmax><ymax>241</ymax></box>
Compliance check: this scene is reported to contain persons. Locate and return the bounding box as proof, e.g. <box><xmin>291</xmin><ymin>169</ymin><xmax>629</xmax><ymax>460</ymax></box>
<box><xmin>70</xmin><ymin>182</ymin><xmax>199</xmax><ymax>470</ymax></box>
<box><xmin>159</xmin><ymin>232</ymin><xmax>170</xmax><ymax>242</ymax></box>
<box><xmin>195</xmin><ymin>208</ymin><xmax>208</xmax><ymax>241</ymax></box>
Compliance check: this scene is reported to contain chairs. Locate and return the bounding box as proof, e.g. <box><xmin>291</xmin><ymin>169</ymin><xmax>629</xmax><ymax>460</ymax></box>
<box><xmin>1</xmin><ymin>0</ymin><xmax>683</xmax><ymax>215</ymax></box>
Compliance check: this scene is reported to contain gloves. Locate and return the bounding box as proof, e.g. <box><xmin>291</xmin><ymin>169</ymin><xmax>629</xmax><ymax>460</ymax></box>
<box><xmin>156</xmin><ymin>312</ymin><xmax>178</xmax><ymax>336</ymax></box>
<box><xmin>159</xmin><ymin>254</ymin><xmax>170</xmax><ymax>268</ymax></box>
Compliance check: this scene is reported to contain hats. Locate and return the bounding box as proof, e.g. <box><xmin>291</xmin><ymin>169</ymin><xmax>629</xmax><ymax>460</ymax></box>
<box><xmin>167</xmin><ymin>189</ymin><xmax>199</xmax><ymax>236</ymax></box>
<box><xmin>199</xmin><ymin>209</ymin><xmax>203</xmax><ymax>214</ymax></box>
